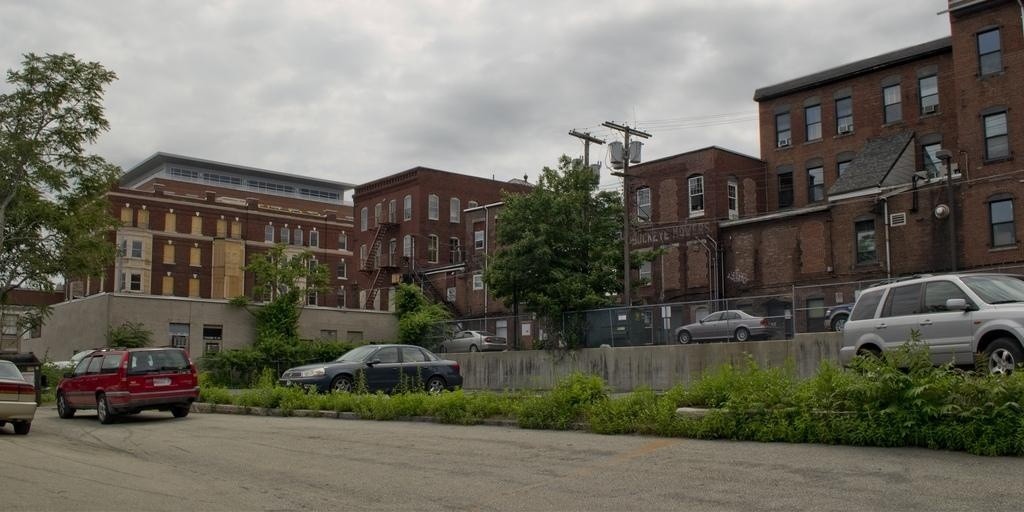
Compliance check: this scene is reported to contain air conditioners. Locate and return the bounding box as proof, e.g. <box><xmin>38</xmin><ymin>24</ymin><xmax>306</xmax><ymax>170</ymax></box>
<box><xmin>924</xmin><ymin>105</ymin><xmax>934</xmax><ymax>113</ymax></box>
<box><xmin>778</xmin><ymin>138</ymin><xmax>787</xmax><ymax>147</ymax></box>
<box><xmin>838</xmin><ymin>124</ymin><xmax>849</xmax><ymax>133</ymax></box>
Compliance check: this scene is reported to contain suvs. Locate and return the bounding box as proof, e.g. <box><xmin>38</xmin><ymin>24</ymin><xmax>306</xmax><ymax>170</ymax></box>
<box><xmin>835</xmin><ymin>273</ymin><xmax>1024</xmax><ymax>380</ymax></box>
<box><xmin>823</xmin><ymin>273</ymin><xmax>934</xmax><ymax>331</ymax></box>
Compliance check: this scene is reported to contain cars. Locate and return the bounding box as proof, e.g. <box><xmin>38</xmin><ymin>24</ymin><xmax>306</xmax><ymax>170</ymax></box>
<box><xmin>437</xmin><ymin>329</ymin><xmax>508</xmax><ymax>352</ymax></box>
<box><xmin>0</xmin><ymin>359</ymin><xmax>38</xmax><ymax>435</ymax></box>
<box><xmin>674</xmin><ymin>311</ymin><xmax>776</xmax><ymax>344</ymax></box>
<box><xmin>278</xmin><ymin>343</ymin><xmax>463</xmax><ymax>396</ymax></box>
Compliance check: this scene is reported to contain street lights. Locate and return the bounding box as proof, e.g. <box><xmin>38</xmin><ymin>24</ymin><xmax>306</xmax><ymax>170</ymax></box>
<box><xmin>935</xmin><ymin>149</ymin><xmax>957</xmax><ymax>272</ymax></box>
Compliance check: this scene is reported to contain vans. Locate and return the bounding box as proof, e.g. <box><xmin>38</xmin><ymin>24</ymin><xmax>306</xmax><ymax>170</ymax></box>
<box><xmin>55</xmin><ymin>346</ymin><xmax>200</xmax><ymax>425</ymax></box>
